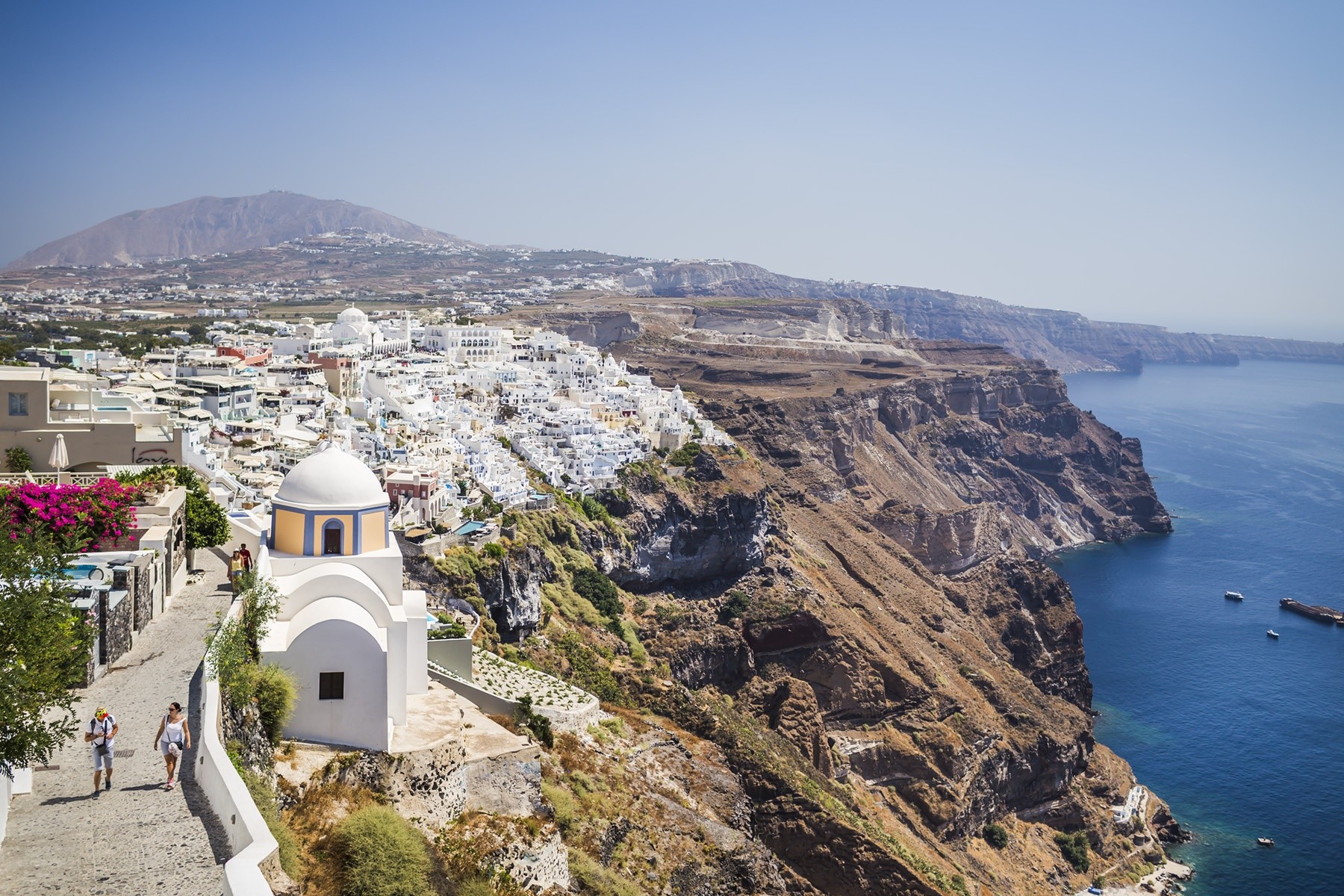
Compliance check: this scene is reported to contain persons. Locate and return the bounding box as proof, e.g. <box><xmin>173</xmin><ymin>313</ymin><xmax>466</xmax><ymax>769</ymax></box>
<box><xmin>154</xmin><ymin>701</ymin><xmax>191</xmax><ymax>790</ymax></box>
<box><xmin>84</xmin><ymin>706</ymin><xmax>118</xmax><ymax>799</ymax></box>
<box><xmin>227</xmin><ymin>548</ymin><xmax>245</xmax><ymax>595</ymax></box>
<box><xmin>239</xmin><ymin>543</ymin><xmax>253</xmax><ymax>573</ymax></box>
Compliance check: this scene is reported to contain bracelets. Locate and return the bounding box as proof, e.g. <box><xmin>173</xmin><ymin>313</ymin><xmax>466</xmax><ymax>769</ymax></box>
<box><xmin>111</xmin><ymin>733</ymin><xmax>114</xmax><ymax>735</ymax></box>
<box><xmin>95</xmin><ymin>733</ymin><xmax>98</xmax><ymax>738</ymax></box>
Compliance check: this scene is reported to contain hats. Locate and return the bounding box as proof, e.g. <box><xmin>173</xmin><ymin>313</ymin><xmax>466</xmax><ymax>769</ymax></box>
<box><xmin>94</xmin><ymin>706</ymin><xmax>108</xmax><ymax>718</ymax></box>
<box><xmin>233</xmin><ymin>548</ymin><xmax>240</xmax><ymax>552</ymax></box>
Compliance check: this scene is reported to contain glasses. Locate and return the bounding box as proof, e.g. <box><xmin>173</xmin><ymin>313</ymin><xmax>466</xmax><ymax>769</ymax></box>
<box><xmin>241</xmin><ymin>546</ymin><xmax>246</xmax><ymax>548</ymax></box>
<box><xmin>169</xmin><ymin>708</ymin><xmax>176</xmax><ymax>712</ymax></box>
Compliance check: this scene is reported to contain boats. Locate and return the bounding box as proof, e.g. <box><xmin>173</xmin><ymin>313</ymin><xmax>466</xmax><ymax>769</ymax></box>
<box><xmin>1224</xmin><ymin>590</ymin><xmax>1244</xmax><ymax>601</ymax></box>
<box><xmin>1267</xmin><ymin>629</ymin><xmax>1280</xmax><ymax>638</ymax></box>
<box><xmin>1279</xmin><ymin>597</ymin><xmax>1344</xmax><ymax>628</ymax></box>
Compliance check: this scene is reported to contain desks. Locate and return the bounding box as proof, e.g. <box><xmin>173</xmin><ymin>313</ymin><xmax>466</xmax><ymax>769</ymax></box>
<box><xmin>108</xmin><ymin>559</ymin><xmax>131</xmax><ymax>568</ymax></box>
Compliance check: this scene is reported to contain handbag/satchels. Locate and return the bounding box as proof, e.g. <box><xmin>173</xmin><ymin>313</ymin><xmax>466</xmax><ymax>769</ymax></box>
<box><xmin>168</xmin><ymin>742</ymin><xmax>183</xmax><ymax>750</ymax></box>
<box><xmin>96</xmin><ymin>744</ymin><xmax>110</xmax><ymax>756</ymax></box>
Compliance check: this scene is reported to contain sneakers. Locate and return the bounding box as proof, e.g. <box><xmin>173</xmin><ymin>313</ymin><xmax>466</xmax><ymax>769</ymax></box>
<box><xmin>167</xmin><ymin>781</ymin><xmax>175</xmax><ymax>789</ymax></box>
<box><xmin>93</xmin><ymin>791</ymin><xmax>100</xmax><ymax>799</ymax></box>
<box><xmin>105</xmin><ymin>776</ymin><xmax>111</xmax><ymax>791</ymax></box>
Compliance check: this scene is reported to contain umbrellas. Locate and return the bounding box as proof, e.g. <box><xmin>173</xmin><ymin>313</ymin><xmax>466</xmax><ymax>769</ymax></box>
<box><xmin>48</xmin><ymin>433</ymin><xmax>69</xmax><ymax>487</ymax></box>
